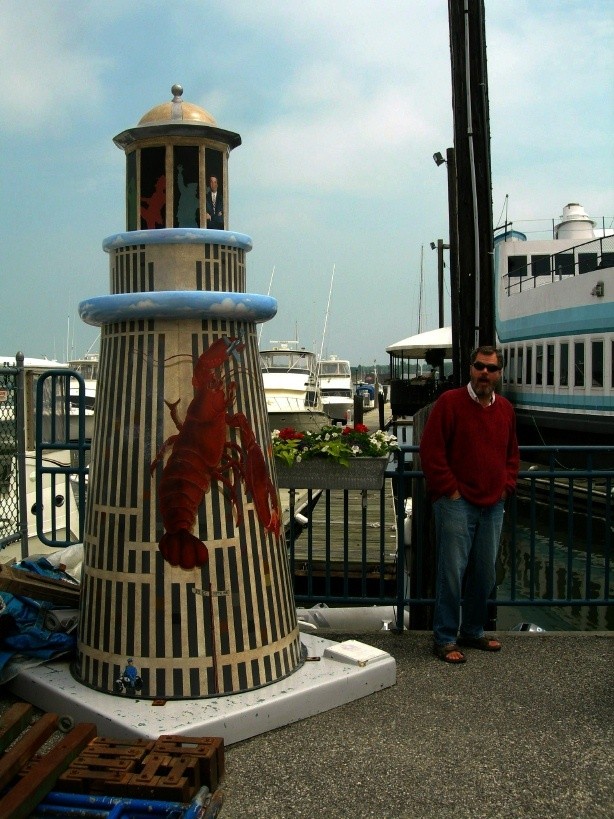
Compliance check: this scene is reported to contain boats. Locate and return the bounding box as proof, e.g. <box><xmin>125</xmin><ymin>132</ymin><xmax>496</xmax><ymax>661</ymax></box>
<box><xmin>252</xmin><ymin>265</ymin><xmax>337</xmax><ymax>435</ymax></box>
<box><xmin>0</xmin><ymin>317</ymin><xmax>102</xmax><ymax>569</ymax></box>
<box><xmin>385</xmin><ymin>194</ymin><xmax>614</xmax><ymax>528</ymax></box>
<box><xmin>353</xmin><ymin>361</ymin><xmax>383</xmax><ymax>412</ymax></box>
<box><xmin>293</xmin><ymin>321</ymin><xmax>354</xmax><ymax>425</ymax></box>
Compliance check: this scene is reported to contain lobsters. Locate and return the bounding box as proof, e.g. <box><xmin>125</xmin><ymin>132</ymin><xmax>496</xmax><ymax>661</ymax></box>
<box><xmin>136</xmin><ymin>336</ymin><xmax>284</xmax><ymax>570</ymax></box>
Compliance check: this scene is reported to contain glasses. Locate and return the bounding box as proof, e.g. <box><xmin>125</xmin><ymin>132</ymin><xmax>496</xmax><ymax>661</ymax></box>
<box><xmin>471</xmin><ymin>361</ymin><xmax>501</xmax><ymax>373</ymax></box>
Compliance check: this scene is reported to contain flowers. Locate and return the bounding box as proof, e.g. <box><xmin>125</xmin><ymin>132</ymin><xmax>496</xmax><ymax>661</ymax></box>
<box><xmin>271</xmin><ymin>424</ymin><xmax>402</xmax><ymax>469</ymax></box>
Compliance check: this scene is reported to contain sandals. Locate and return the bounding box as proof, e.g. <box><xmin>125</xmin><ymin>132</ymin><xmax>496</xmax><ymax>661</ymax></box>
<box><xmin>456</xmin><ymin>636</ymin><xmax>502</xmax><ymax>651</ymax></box>
<box><xmin>433</xmin><ymin>643</ymin><xmax>467</xmax><ymax>663</ymax></box>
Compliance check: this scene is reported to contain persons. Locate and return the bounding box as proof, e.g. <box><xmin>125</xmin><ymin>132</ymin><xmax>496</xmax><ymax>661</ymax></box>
<box><xmin>421</xmin><ymin>346</ymin><xmax>521</xmax><ymax>663</ymax></box>
<box><xmin>430</xmin><ymin>368</ymin><xmax>439</xmax><ymax>390</ymax></box>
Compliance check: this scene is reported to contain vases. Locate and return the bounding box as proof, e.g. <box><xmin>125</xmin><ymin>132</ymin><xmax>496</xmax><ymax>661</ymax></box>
<box><xmin>275</xmin><ymin>456</ymin><xmax>389</xmax><ymax>490</ymax></box>
